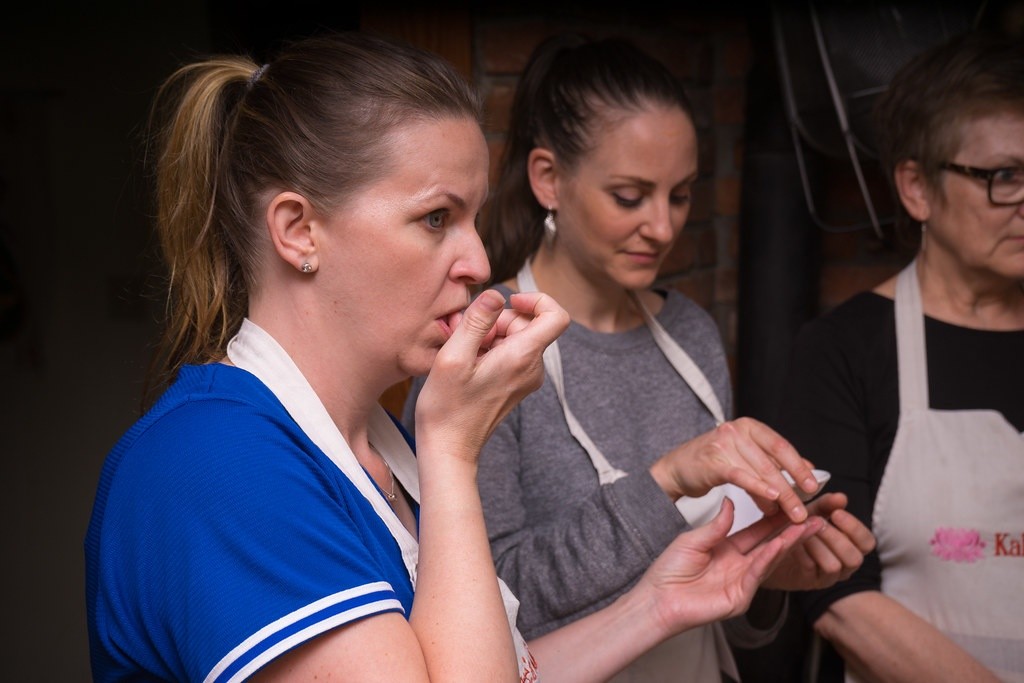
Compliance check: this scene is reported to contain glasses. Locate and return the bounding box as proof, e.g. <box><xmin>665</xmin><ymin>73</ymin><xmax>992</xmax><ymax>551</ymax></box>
<box><xmin>913</xmin><ymin>160</ymin><xmax>1024</xmax><ymax>207</ymax></box>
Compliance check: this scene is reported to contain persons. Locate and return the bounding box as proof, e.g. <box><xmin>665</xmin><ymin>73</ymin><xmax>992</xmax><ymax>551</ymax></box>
<box><xmin>84</xmin><ymin>30</ymin><xmax>823</xmax><ymax>683</ymax></box>
<box><xmin>771</xmin><ymin>28</ymin><xmax>1024</xmax><ymax>683</ymax></box>
<box><xmin>401</xmin><ymin>25</ymin><xmax>876</xmax><ymax>683</ymax></box>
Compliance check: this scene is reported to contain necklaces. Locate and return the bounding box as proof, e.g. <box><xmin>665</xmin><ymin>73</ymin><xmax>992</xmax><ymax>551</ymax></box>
<box><xmin>367</xmin><ymin>445</ymin><xmax>396</xmax><ymax>501</ymax></box>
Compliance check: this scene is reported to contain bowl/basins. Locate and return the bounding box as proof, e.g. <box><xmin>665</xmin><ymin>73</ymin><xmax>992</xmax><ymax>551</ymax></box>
<box><xmin>774</xmin><ymin>468</ymin><xmax>831</xmax><ymax>504</ymax></box>
